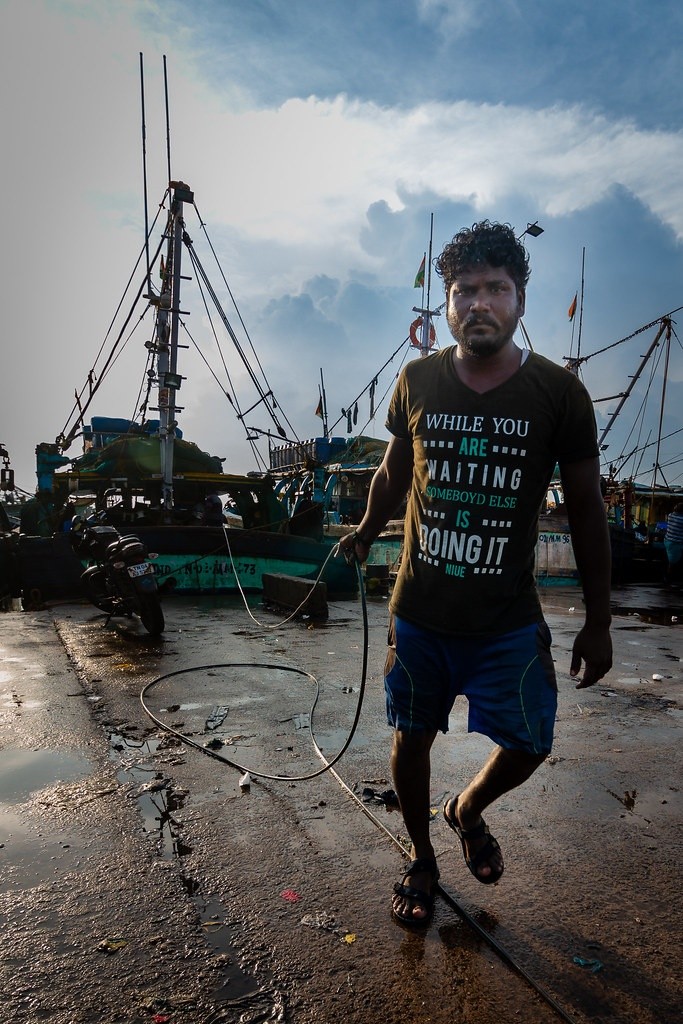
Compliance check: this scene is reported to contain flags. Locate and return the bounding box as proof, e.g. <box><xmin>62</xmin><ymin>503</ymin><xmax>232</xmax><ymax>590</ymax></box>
<box><xmin>568</xmin><ymin>294</ymin><xmax>577</xmax><ymax>322</ymax></box>
<box><xmin>414</xmin><ymin>252</ymin><xmax>426</xmax><ymax>290</ymax></box>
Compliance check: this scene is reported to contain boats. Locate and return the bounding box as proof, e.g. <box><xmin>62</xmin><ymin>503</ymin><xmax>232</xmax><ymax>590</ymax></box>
<box><xmin>0</xmin><ymin>40</ymin><xmax>683</xmax><ymax>604</ymax></box>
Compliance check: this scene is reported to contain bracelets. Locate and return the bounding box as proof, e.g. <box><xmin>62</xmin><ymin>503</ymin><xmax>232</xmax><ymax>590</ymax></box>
<box><xmin>351</xmin><ymin>529</ymin><xmax>365</xmax><ymax>543</ymax></box>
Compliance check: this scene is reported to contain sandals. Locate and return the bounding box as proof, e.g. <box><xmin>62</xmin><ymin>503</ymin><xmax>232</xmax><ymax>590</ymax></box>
<box><xmin>443</xmin><ymin>792</ymin><xmax>505</xmax><ymax>884</ymax></box>
<box><xmin>391</xmin><ymin>854</ymin><xmax>441</xmax><ymax>924</ymax></box>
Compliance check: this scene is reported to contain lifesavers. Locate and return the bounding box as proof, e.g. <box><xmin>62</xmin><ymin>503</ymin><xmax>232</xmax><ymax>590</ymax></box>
<box><xmin>30</xmin><ymin>588</ymin><xmax>42</xmax><ymax>603</ymax></box>
<box><xmin>339</xmin><ymin>515</ymin><xmax>354</xmax><ymax>525</ymax></box>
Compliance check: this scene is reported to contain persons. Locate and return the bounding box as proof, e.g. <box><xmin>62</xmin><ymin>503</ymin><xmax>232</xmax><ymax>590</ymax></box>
<box><xmin>21</xmin><ymin>488</ymin><xmax>62</xmax><ymax>538</ymax></box>
<box><xmin>339</xmin><ymin>220</ymin><xmax>614</xmax><ymax>929</ymax></box>
<box><xmin>663</xmin><ymin>504</ymin><xmax>683</xmax><ymax>588</ymax></box>
